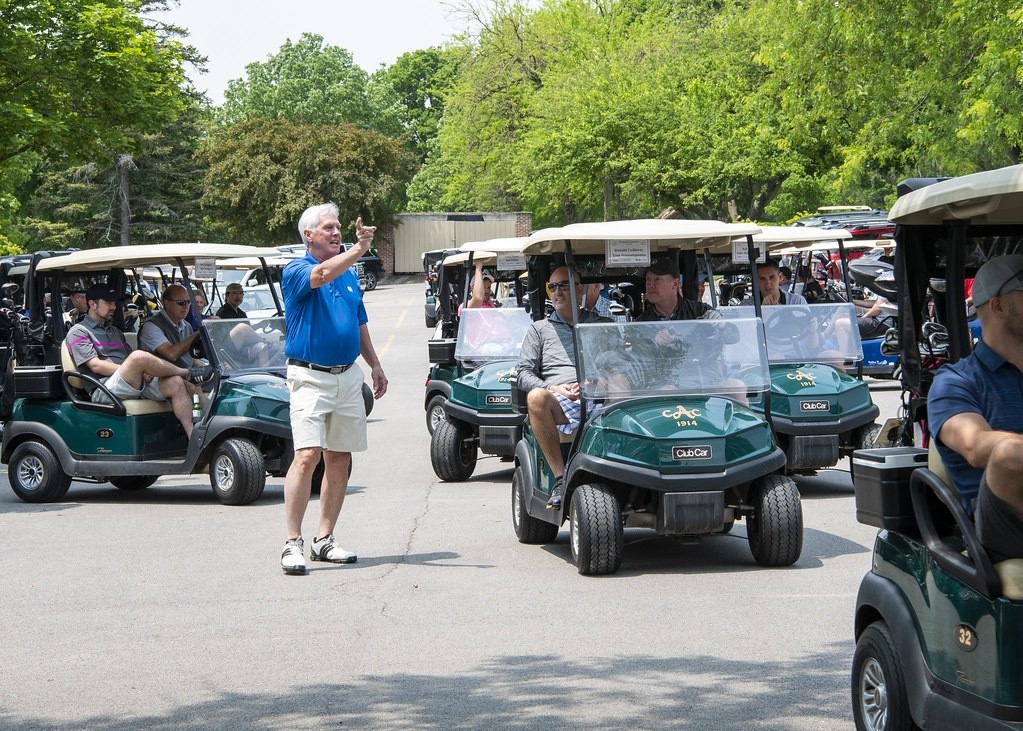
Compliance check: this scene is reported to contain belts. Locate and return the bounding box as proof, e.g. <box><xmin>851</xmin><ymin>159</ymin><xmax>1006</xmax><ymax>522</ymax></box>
<box><xmin>289</xmin><ymin>359</ymin><xmax>353</xmax><ymax>375</ymax></box>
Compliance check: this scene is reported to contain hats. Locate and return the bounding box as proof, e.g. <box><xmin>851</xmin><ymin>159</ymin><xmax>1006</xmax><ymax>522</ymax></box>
<box><xmin>971</xmin><ymin>254</ymin><xmax>1023</xmax><ymax>308</ymax></box>
<box><xmin>225</xmin><ymin>283</ymin><xmax>247</xmax><ymax>293</ymax></box>
<box><xmin>640</xmin><ymin>261</ymin><xmax>678</xmax><ymax>276</ymax></box>
<box><xmin>86</xmin><ymin>284</ymin><xmax>123</xmax><ymax>302</ymax></box>
<box><xmin>469</xmin><ymin>273</ymin><xmax>495</xmax><ymax>288</ymax></box>
<box><xmin>678</xmin><ymin>273</ymin><xmax>709</xmax><ymax>286</ymax></box>
<box><xmin>62</xmin><ymin>285</ymin><xmax>86</xmax><ymax>293</ymax></box>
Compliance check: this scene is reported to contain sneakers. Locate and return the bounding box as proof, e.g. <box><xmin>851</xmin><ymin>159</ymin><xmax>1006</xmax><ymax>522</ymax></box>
<box><xmin>310</xmin><ymin>536</ymin><xmax>358</xmax><ymax>565</ymax></box>
<box><xmin>187</xmin><ymin>364</ymin><xmax>214</xmax><ymax>386</ymax></box>
<box><xmin>282</xmin><ymin>536</ymin><xmax>306</xmax><ymax>575</ymax></box>
<box><xmin>547</xmin><ymin>480</ymin><xmax>562</xmax><ymax>510</ymax></box>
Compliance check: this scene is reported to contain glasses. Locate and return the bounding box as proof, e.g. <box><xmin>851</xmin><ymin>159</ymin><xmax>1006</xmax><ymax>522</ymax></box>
<box><xmin>548</xmin><ymin>281</ymin><xmax>579</xmax><ymax>292</ymax></box>
<box><xmin>168</xmin><ymin>299</ymin><xmax>191</xmax><ymax>306</ymax></box>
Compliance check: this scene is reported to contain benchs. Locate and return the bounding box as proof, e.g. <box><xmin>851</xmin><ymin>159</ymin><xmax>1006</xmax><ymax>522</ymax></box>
<box><xmin>789</xmin><ymin>283</ymin><xmax>805</xmax><ymax>295</ymax></box>
<box><xmin>910</xmin><ymin>434</ymin><xmax>1023</xmax><ymax>601</ymax></box>
<box><xmin>61</xmin><ymin>333</ymin><xmax>174</xmax><ymax>416</ymax></box>
<box><xmin>861</xmin><ymin>316</ymin><xmax>898</xmax><ymax>340</ymax></box>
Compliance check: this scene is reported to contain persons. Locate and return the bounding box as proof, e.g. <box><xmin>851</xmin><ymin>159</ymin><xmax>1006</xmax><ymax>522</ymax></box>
<box><xmin>281</xmin><ymin>202</ymin><xmax>389</xmax><ymax>575</ymax></box>
<box><xmin>457</xmin><ymin>247</ymin><xmax>896</xmax><ymax>512</ymax></box>
<box><xmin>929</xmin><ymin>254</ymin><xmax>1023</xmax><ymax>561</ymax></box>
<box><xmin>20</xmin><ymin>282</ymin><xmax>280</xmax><ymax>443</ymax></box>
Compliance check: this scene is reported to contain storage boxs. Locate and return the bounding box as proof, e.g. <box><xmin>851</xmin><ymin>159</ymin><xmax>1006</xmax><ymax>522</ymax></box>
<box><xmin>853</xmin><ymin>446</ymin><xmax>928</xmax><ymax>529</ymax></box>
<box><xmin>428</xmin><ymin>338</ymin><xmax>456</xmax><ymax>363</ymax></box>
<box><xmin>508</xmin><ymin>375</ymin><xmax>528</xmax><ymax>413</ymax></box>
<box><xmin>14</xmin><ymin>365</ymin><xmax>66</xmax><ymax>398</ymax></box>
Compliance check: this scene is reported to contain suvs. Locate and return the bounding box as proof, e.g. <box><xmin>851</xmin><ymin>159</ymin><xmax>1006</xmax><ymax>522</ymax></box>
<box><xmin>338</xmin><ymin>242</ymin><xmax>386</xmax><ymax>290</ymax></box>
